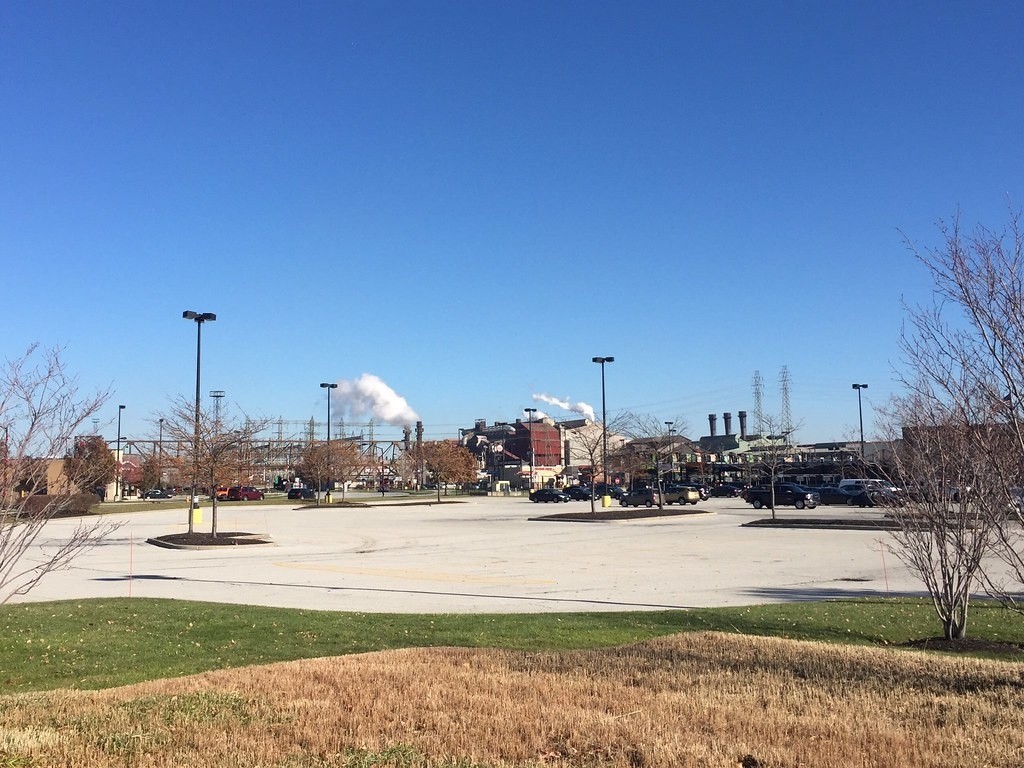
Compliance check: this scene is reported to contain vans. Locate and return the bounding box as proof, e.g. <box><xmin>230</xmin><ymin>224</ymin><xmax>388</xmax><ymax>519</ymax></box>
<box><xmin>840</xmin><ymin>479</ymin><xmax>903</xmax><ymax>496</ymax></box>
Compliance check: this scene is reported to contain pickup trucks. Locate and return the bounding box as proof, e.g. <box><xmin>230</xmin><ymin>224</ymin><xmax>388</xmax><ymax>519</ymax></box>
<box><xmin>906</xmin><ymin>480</ymin><xmax>971</xmax><ymax>503</ymax></box>
<box><xmin>747</xmin><ymin>484</ymin><xmax>821</xmax><ymax>509</ymax></box>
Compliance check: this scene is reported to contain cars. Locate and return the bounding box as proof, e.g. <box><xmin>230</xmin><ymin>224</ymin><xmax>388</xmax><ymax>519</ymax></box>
<box><xmin>851</xmin><ymin>489</ymin><xmax>907</xmax><ymax>508</ymax></box>
<box><xmin>710</xmin><ymin>485</ymin><xmax>743</xmax><ymax>498</ymax></box>
<box><xmin>529</xmin><ymin>488</ymin><xmax>571</xmax><ymax>503</ymax></box>
<box><xmin>470</xmin><ymin>482</ymin><xmax>479</xmax><ymax>490</ymax></box>
<box><xmin>440</xmin><ymin>482</ymin><xmax>456</xmax><ymax>490</ymax></box>
<box><xmin>568</xmin><ymin>488</ymin><xmax>599</xmax><ymax>501</ymax></box>
<box><xmin>569</xmin><ymin>483</ymin><xmax>621</xmax><ymax>498</ymax></box>
<box><xmin>215</xmin><ymin>487</ymin><xmax>231</xmax><ymax>500</ymax></box>
<box><xmin>378</xmin><ymin>485</ymin><xmax>389</xmax><ymax>492</ymax></box>
<box><xmin>814</xmin><ymin>488</ymin><xmax>858</xmax><ymax>505</ymax></box>
<box><xmin>139</xmin><ymin>490</ymin><xmax>172</xmax><ymax>498</ymax></box>
<box><xmin>1005</xmin><ymin>487</ymin><xmax>1024</xmax><ymax>517</ymax></box>
<box><xmin>423</xmin><ymin>484</ymin><xmax>436</xmax><ymax>490</ymax></box>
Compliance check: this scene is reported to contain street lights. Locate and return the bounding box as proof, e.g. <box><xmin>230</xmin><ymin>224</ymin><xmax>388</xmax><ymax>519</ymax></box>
<box><xmin>524</xmin><ymin>408</ymin><xmax>537</xmax><ymax>489</ymax></box>
<box><xmin>158</xmin><ymin>419</ymin><xmax>164</xmax><ymax>489</ymax></box>
<box><xmin>592</xmin><ymin>356</ymin><xmax>614</xmax><ymax>509</ymax></box>
<box><xmin>320</xmin><ymin>383</ymin><xmax>338</xmax><ymax>504</ymax></box>
<box><xmin>182</xmin><ymin>310</ymin><xmax>216</xmax><ymax>534</ymax></box>
<box><xmin>114</xmin><ymin>405</ymin><xmax>125</xmax><ymax>501</ymax></box>
<box><xmin>853</xmin><ymin>384</ymin><xmax>868</xmax><ymax>478</ymax></box>
<box><xmin>495</xmin><ymin>422</ymin><xmax>507</xmax><ymax>481</ymax></box>
<box><xmin>665</xmin><ymin>422</ymin><xmax>673</xmax><ymax>468</ymax></box>
<box><xmin>670</xmin><ymin>429</ymin><xmax>676</xmax><ymax>455</ymax></box>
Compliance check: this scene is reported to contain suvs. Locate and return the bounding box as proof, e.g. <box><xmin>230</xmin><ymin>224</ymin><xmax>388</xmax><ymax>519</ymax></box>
<box><xmin>619</xmin><ymin>489</ymin><xmax>666</xmax><ymax>507</ymax></box>
<box><xmin>664</xmin><ymin>487</ymin><xmax>700</xmax><ymax>506</ymax></box>
<box><xmin>229</xmin><ymin>486</ymin><xmax>264</xmax><ymax>501</ymax></box>
<box><xmin>681</xmin><ymin>485</ymin><xmax>712</xmax><ymax>501</ymax></box>
<box><xmin>288</xmin><ymin>488</ymin><xmax>316</xmax><ymax>500</ymax></box>
